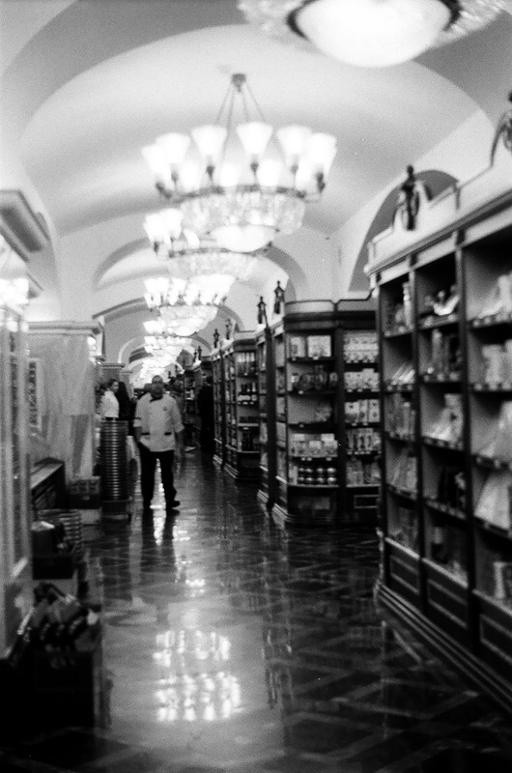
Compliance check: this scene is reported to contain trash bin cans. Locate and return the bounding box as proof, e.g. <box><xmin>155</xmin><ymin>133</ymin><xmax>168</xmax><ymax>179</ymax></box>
<box><xmin>37</xmin><ymin>508</ymin><xmax>82</xmax><ymax>561</ymax></box>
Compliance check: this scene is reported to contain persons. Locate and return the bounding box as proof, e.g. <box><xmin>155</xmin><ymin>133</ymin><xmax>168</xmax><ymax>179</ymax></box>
<box><xmin>117</xmin><ymin>381</ymin><xmax>137</xmax><ymax>434</ymax></box>
<box><xmin>196</xmin><ymin>375</ymin><xmax>213</xmax><ymax>465</ymax></box>
<box><xmin>96</xmin><ymin>377</ymin><xmax>122</xmax><ymax>420</ymax></box>
<box><xmin>133</xmin><ymin>373</ymin><xmax>187</xmax><ymax>508</ymax></box>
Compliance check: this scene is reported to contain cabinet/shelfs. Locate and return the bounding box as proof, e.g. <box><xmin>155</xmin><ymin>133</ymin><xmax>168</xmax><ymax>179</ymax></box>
<box><xmin>169</xmin><ymin>91</ymin><xmax>512</xmax><ymax>714</ymax></box>
<box><xmin>1</xmin><ymin>188</ymin><xmax>49</xmax><ymax>655</ymax></box>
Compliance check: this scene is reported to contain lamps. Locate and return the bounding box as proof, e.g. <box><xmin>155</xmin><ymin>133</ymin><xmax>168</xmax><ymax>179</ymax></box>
<box><xmin>236</xmin><ymin>1</ymin><xmax>506</xmax><ymax>72</ymax></box>
<box><xmin>144</xmin><ymin>69</ymin><xmax>339</xmax><ymax>256</ymax></box>
<box><xmin>145</xmin><ymin>202</ymin><xmax>266</xmax><ymax>369</ymax></box>
